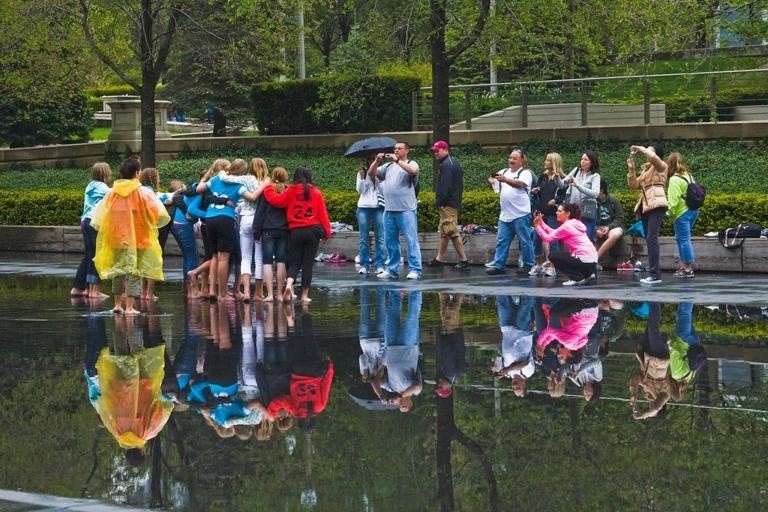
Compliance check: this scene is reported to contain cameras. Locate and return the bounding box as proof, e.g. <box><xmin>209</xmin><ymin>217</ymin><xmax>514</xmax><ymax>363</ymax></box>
<box><xmin>383</xmin><ymin>398</ymin><xmax>401</xmax><ymax>407</ymax></box>
<box><xmin>382</xmin><ymin>152</ymin><xmax>393</xmax><ymax>160</ymax></box>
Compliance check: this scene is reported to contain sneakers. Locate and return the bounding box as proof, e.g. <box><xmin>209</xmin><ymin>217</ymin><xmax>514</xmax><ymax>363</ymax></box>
<box><xmin>485</xmin><ymin>267</ymin><xmax>506</xmax><ymax>275</ymax></box>
<box><xmin>545</xmin><ymin>266</ymin><xmax>556</xmax><ymax>277</ymax></box>
<box><xmin>640</xmin><ymin>276</ymin><xmax>663</xmax><ymax>285</ymax></box>
<box><xmin>617</xmin><ymin>262</ymin><xmax>633</xmax><ymax>270</ymax></box>
<box><xmin>515</xmin><ymin>266</ymin><xmax>531</xmax><ymax>275</ymax></box>
<box><xmin>377</xmin><ymin>270</ymin><xmax>399</xmax><ymax>280</ymax></box>
<box><xmin>358</xmin><ymin>265</ymin><xmax>369</xmax><ymax>274</ymax></box>
<box><xmin>673</xmin><ymin>266</ymin><xmax>696</xmax><ymax>278</ymax></box>
<box><xmin>585</xmin><ymin>273</ymin><xmax>596</xmax><ymax>283</ymax></box>
<box><xmin>634</xmin><ymin>260</ymin><xmax>645</xmax><ymax>272</ymax></box>
<box><xmin>314</xmin><ymin>253</ymin><xmax>347</xmax><ymax>263</ymax></box>
<box><xmin>528</xmin><ymin>264</ymin><xmax>540</xmax><ymax>276</ymax></box>
<box><xmin>562</xmin><ymin>276</ymin><xmax>585</xmax><ymax>285</ymax></box>
<box><xmin>427</xmin><ymin>259</ymin><xmax>445</xmax><ymax>268</ymax></box>
<box><xmin>407</xmin><ymin>270</ymin><xmax>422</xmax><ymax>281</ymax></box>
<box><xmin>376</xmin><ymin>266</ymin><xmax>384</xmax><ymax>274</ymax></box>
<box><xmin>453</xmin><ymin>260</ymin><xmax>471</xmax><ymax>271</ymax></box>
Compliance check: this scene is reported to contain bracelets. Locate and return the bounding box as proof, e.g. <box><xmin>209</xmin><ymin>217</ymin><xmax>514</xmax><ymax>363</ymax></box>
<box><xmin>394</xmin><ymin>158</ymin><xmax>400</xmax><ymax>164</ymax></box>
<box><xmin>398</xmin><ymin>392</ymin><xmax>404</xmax><ymax>398</ymax></box>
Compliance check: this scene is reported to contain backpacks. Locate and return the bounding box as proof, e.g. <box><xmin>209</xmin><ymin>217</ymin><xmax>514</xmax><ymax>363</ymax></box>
<box><xmin>719</xmin><ymin>222</ymin><xmax>762</xmax><ymax>240</ymax></box>
<box><xmin>682</xmin><ymin>344</ymin><xmax>709</xmax><ymax>373</ymax></box>
<box><xmin>414</xmin><ymin>181</ymin><xmax>421</xmax><ymax>198</ymax></box>
<box><xmin>686</xmin><ymin>182</ymin><xmax>705</xmax><ymax>210</ymax></box>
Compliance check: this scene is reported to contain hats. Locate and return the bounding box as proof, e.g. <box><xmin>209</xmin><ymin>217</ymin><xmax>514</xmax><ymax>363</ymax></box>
<box><xmin>430</xmin><ymin>141</ymin><xmax>448</xmax><ymax>152</ymax></box>
<box><xmin>434</xmin><ymin>387</ymin><xmax>453</xmax><ymax>399</ymax></box>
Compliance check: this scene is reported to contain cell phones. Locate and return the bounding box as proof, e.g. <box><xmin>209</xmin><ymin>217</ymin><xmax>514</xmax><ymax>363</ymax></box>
<box><xmin>533</xmin><ymin>210</ymin><xmax>542</xmax><ymax>219</ymax></box>
<box><xmin>493</xmin><ymin>370</ymin><xmax>504</xmax><ymax>378</ymax></box>
<box><xmin>492</xmin><ymin>172</ymin><xmax>503</xmax><ymax>180</ymax></box>
<box><xmin>628</xmin><ymin>149</ymin><xmax>637</xmax><ymax>162</ymax></box>
<box><xmin>533</xmin><ymin>357</ymin><xmax>543</xmax><ymax>368</ymax></box>
<box><xmin>633</xmin><ymin>405</ymin><xmax>640</xmax><ymax>416</ymax></box>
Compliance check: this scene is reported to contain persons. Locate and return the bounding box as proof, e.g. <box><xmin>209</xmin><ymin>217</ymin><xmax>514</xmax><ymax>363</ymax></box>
<box><xmin>666</xmin><ymin>151</ymin><xmax>705</xmax><ymax>278</ymax></box>
<box><xmin>174</xmin><ymin>300</ymin><xmax>334</xmax><ymax>441</ymax></box>
<box><xmin>355</xmin><ymin>150</ymin><xmax>385</xmax><ymax>274</ymax></box>
<box><xmin>79</xmin><ymin>314</ymin><xmax>189</xmax><ymax>468</ymax></box>
<box><xmin>424</xmin><ymin>140</ymin><xmax>470</xmax><ymax>269</ymax></box>
<box><xmin>432</xmin><ymin>293</ymin><xmax>467</xmax><ymax>398</ymax></box>
<box><xmin>593</xmin><ymin>181</ymin><xmax>626</xmax><ymax>270</ymax></box>
<box><xmin>357</xmin><ymin>285</ymin><xmax>387</xmax><ymax>384</ymax></box>
<box><xmin>483</xmin><ymin>146</ymin><xmax>602</xmax><ymax>285</ymax></box>
<box><xmin>370</xmin><ymin>290</ymin><xmax>423</xmax><ymax>412</ymax></box>
<box><xmin>667</xmin><ymin>303</ymin><xmax>709</xmax><ymax>403</ymax></box>
<box><xmin>489</xmin><ymin>296</ymin><xmax>629</xmax><ymax>405</ymax></box>
<box><xmin>368</xmin><ymin>141</ymin><xmax>423</xmax><ymax>280</ymax></box>
<box><xmin>73</xmin><ymin>162</ymin><xmax>185</xmax><ymax>300</ymax></box>
<box><xmin>629</xmin><ymin>302</ymin><xmax>671</xmax><ymax>420</ymax></box>
<box><xmin>90</xmin><ymin>156</ymin><xmax>170</xmax><ymax>314</ymax></box>
<box><xmin>625</xmin><ymin>143</ymin><xmax>669</xmax><ymax>284</ymax></box>
<box><xmin>174</xmin><ymin>158</ymin><xmax>333</xmax><ymax>300</ymax></box>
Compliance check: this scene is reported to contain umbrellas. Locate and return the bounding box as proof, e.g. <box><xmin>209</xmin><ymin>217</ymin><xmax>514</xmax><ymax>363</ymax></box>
<box><xmin>342</xmin><ymin>135</ymin><xmax>398</xmax><ymax>158</ymax></box>
<box><xmin>349</xmin><ymin>387</ymin><xmax>396</xmax><ymax>412</ymax></box>
